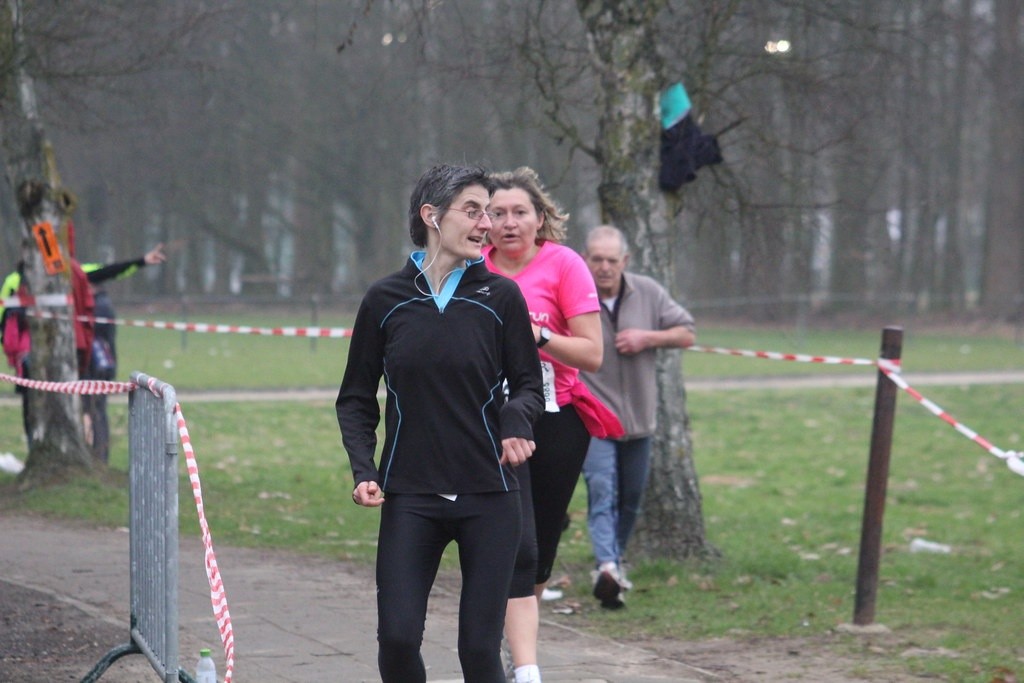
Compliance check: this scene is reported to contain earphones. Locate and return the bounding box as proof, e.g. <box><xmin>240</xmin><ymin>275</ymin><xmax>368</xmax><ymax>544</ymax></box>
<box><xmin>431</xmin><ymin>216</ymin><xmax>439</xmax><ymax>229</ymax></box>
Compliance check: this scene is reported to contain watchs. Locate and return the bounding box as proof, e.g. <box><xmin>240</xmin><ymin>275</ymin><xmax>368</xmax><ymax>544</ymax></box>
<box><xmin>536</xmin><ymin>327</ymin><xmax>552</xmax><ymax>347</ymax></box>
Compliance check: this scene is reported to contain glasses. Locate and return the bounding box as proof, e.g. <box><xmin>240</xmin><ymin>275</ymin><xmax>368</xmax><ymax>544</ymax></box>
<box><xmin>440</xmin><ymin>206</ymin><xmax>496</xmax><ymax>222</ymax></box>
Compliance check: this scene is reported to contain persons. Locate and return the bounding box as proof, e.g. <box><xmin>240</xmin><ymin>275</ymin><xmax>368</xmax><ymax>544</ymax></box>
<box><xmin>1</xmin><ymin>229</ymin><xmax>168</xmax><ymax>468</ymax></box>
<box><xmin>478</xmin><ymin>166</ymin><xmax>603</xmax><ymax>683</ymax></box>
<box><xmin>577</xmin><ymin>224</ymin><xmax>696</xmax><ymax>614</ymax></box>
<box><xmin>336</xmin><ymin>162</ymin><xmax>545</xmax><ymax>683</ymax></box>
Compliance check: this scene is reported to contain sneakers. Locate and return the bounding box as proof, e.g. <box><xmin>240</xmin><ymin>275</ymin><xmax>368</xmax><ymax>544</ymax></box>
<box><xmin>592</xmin><ymin>565</ymin><xmax>624</xmax><ymax>608</ymax></box>
